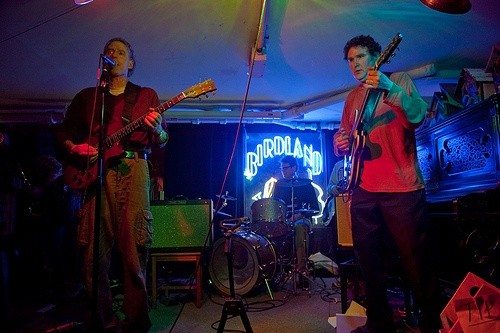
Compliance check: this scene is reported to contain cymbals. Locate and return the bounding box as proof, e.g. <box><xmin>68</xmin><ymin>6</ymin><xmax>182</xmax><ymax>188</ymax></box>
<box><xmin>289</xmin><ymin>209</ymin><xmax>320</xmax><ymax>216</ymax></box>
<box><xmin>277</xmin><ymin>178</ymin><xmax>312</xmax><ymax>187</ymax></box>
<box><xmin>216</xmin><ymin>195</ymin><xmax>238</xmax><ymax>201</ymax></box>
<box><xmin>214</xmin><ymin>209</ymin><xmax>232</xmax><ymax>218</ymax></box>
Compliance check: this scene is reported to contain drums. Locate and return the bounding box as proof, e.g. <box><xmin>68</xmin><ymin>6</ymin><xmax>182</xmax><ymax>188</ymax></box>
<box><xmin>269</xmin><ymin>236</ymin><xmax>292</xmax><ymax>265</ymax></box>
<box><xmin>208</xmin><ymin>231</ymin><xmax>277</xmax><ymax>297</ymax></box>
<box><xmin>251</xmin><ymin>198</ymin><xmax>289</xmax><ymax>236</ymax></box>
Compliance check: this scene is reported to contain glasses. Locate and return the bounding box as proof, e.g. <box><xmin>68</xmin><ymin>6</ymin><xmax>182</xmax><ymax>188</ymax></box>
<box><xmin>279</xmin><ymin>165</ymin><xmax>293</xmax><ymax>170</ymax></box>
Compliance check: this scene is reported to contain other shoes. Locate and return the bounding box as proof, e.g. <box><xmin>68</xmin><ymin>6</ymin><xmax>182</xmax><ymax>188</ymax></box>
<box><xmin>298</xmin><ymin>271</ymin><xmax>309</xmax><ymax>288</ymax></box>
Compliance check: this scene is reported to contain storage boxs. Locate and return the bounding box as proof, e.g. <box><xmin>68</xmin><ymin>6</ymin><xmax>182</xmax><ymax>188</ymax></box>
<box><xmin>336</xmin><ymin>193</ymin><xmax>353</xmax><ymax>245</ymax></box>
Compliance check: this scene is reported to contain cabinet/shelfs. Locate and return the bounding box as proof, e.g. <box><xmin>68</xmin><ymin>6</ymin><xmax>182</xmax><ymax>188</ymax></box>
<box><xmin>414</xmin><ymin>96</ymin><xmax>500</xmax><ymax>201</ymax></box>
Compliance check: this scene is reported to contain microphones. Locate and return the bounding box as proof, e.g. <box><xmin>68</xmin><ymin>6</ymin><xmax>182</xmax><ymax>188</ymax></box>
<box><xmin>100</xmin><ymin>54</ymin><xmax>117</xmax><ymax>68</ymax></box>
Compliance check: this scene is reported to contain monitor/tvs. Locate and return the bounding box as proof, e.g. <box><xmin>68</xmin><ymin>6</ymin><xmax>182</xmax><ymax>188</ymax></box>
<box><xmin>137</xmin><ymin>199</ymin><xmax>214</xmax><ymax>253</ymax></box>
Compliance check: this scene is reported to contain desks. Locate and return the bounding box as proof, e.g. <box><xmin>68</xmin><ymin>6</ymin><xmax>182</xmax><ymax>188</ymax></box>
<box><xmin>341</xmin><ymin>258</ymin><xmax>414</xmax><ymax>317</ymax></box>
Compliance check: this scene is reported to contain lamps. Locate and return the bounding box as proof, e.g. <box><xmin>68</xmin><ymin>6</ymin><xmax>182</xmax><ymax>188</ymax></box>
<box><xmin>74</xmin><ymin>0</ymin><xmax>96</xmax><ymax>6</ymax></box>
<box><xmin>420</xmin><ymin>0</ymin><xmax>473</xmax><ymax>15</ymax></box>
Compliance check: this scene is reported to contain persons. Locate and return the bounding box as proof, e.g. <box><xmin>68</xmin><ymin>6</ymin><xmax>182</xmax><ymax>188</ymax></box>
<box><xmin>333</xmin><ymin>35</ymin><xmax>444</xmax><ymax>333</ymax></box>
<box><xmin>56</xmin><ymin>38</ymin><xmax>169</xmax><ymax>333</ymax></box>
<box><xmin>271</xmin><ymin>156</ymin><xmax>319</xmax><ymax>266</ymax></box>
<box><xmin>328</xmin><ymin>159</ymin><xmax>354</xmax><ymax>287</ymax></box>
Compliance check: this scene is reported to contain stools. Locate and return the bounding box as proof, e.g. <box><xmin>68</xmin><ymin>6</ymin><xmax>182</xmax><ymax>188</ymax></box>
<box><xmin>152</xmin><ymin>252</ymin><xmax>201</xmax><ymax>308</ymax></box>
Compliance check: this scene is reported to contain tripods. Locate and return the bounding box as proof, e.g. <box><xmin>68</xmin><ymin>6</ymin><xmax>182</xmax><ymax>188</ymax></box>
<box><xmin>271</xmin><ymin>184</ymin><xmax>331</xmax><ymax>299</ymax></box>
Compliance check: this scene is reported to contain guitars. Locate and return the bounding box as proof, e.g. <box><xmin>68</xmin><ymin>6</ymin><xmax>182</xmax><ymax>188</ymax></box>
<box><xmin>64</xmin><ymin>77</ymin><xmax>217</xmax><ymax>190</ymax></box>
<box><xmin>343</xmin><ymin>33</ymin><xmax>403</xmax><ymax>191</ymax></box>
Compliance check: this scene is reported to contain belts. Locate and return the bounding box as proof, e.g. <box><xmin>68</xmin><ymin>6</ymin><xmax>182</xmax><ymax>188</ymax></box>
<box><xmin>122</xmin><ymin>151</ymin><xmax>149</xmax><ymax>159</ymax></box>
